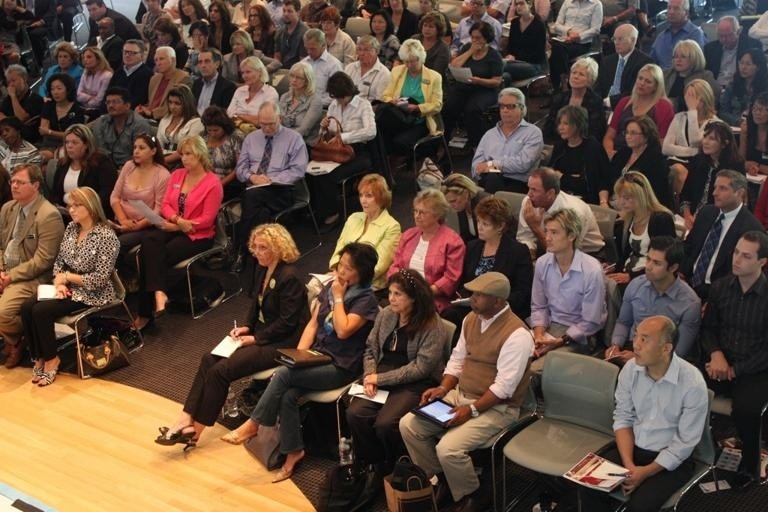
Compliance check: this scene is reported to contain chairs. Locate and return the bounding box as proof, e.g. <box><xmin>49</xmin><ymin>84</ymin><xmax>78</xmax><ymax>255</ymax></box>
<box><xmin>444</xmin><ymin>144</ymin><xmax>617</xmax><ymax>261</ymax></box>
<box><xmin>505</xmin><ymin>34</ymin><xmax>604</xmax><ymax>129</ymax></box>
<box><xmin>640</xmin><ymin>3</ymin><xmax>762</xmax><ymax>43</ymax></box>
<box><xmin>250</xmin><ymin>316</ymin><xmax>725</xmax><ymax>509</ymax></box>
<box><xmin>21</xmin><ymin>2</ymin><xmax>89</xmax><ymax>91</ymax></box>
<box><xmin>56</xmin><ymin>195</ymin><xmax>245</xmax><ymax>381</ymax></box>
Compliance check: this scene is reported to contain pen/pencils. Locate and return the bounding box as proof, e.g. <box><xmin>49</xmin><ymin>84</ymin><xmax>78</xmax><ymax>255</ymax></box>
<box><xmin>609</xmin><ymin>344</ymin><xmax>616</xmax><ymax>358</ymax></box>
<box><xmin>139</xmin><ymin>103</ymin><xmax>141</xmax><ymax>112</ymax></box>
<box><xmin>456</xmin><ymin>291</ymin><xmax>462</xmax><ymax>299</ymax></box>
<box><xmin>354</xmin><ymin>393</ymin><xmax>364</xmax><ymax>395</ymax></box>
<box><xmin>607</xmin><ymin>474</ymin><xmax>625</xmax><ymax>475</ymax></box>
<box><xmin>604</xmin><ymin>263</ymin><xmax>617</xmax><ymax>271</ymax></box>
<box><xmin>59</xmin><ymin>289</ymin><xmax>77</xmax><ymax>292</ymax></box>
<box><xmin>234</xmin><ymin>320</ymin><xmax>237</xmax><ymax>334</ymax></box>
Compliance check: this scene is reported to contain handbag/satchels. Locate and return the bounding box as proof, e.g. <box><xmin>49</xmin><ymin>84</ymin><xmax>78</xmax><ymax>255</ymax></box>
<box><xmin>314</xmin><ymin>462</ymin><xmax>378</xmax><ymax>512</ymax></box>
<box><xmin>308</xmin><ymin>115</ymin><xmax>357</xmax><ymax>163</ymax></box>
<box><xmin>382</xmin><ymin>454</ymin><xmax>439</xmax><ymax>512</ymax></box>
<box><xmin>414</xmin><ymin>156</ymin><xmax>446</xmax><ymax>199</ymax></box>
<box><xmin>73</xmin><ymin>333</ymin><xmax>132</xmax><ymax>377</ymax></box>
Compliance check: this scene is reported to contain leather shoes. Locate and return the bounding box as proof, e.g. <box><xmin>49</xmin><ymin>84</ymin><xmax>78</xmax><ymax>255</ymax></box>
<box><xmin>432</xmin><ymin>480</ymin><xmax>486</xmax><ymax>512</ymax></box>
<box><xmin>732</xmin><ymin>460</ymin><xmax>758</xmax><ymax>492</ymax></box>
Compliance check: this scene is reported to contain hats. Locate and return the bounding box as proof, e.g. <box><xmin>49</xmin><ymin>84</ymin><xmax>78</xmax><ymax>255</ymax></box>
<box><xmin>461</xmin><ymin>269</ymin><xmax>512</xmax><ymax>300</ymax></box>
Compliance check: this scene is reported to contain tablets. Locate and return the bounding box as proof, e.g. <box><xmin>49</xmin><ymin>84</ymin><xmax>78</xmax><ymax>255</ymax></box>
<box><xmin>411</xmin><ymin>397</ymin><xmax>459</xmax><ymax>428</ymax></box>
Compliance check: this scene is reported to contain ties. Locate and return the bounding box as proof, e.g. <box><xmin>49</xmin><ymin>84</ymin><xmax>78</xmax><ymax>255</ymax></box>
<box><xmin>4</xmin><ymin>207</ymin><xmax>29</xmax><ymax>275</ymax></box>
<box><xmin>691</xmin><ymin>211</ymin><xmax>732</xmax><ymax>289</ymax></box>
<box><xmin>610</xmin><ymin>58</ymin><xmax>627</xmax><ymax>100</ymax></box>
<box><xmin>257</xmin><ymin>135</ymin><xmax>275</xmax><ymax>182</ymax></box>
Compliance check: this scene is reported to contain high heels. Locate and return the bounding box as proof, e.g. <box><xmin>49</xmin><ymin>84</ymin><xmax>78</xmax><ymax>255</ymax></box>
<box><xmin>30</xmin><ymin>356</ymin><xmax>46</xmax><ymax>384</ymax></box>
<box><xmin>220</xmin><ymin>428</ymin><xmax>259</xmax><ymax>446</ymax></box>
<box><xmin>37</xmin><ymin>354</ymin><xmax>61</xmax><ymax>386</ymax></box>
<box><xmin>125</xmin><ymin>312</ymin><xmax>154</xmax><ymax>336</ymax></box>
<box><xmin>151</xmin><ymin>292</ymin><xmax>171</xmax><ymax>319</ymax></box>
<box><xmin>154</xmin><ymin>422</ymin><xmax>199</xmax><ymax>452</ymax></box>
<box><xmin>271</xmin><ymin>448</ymin><xmax>305</xmax><ymax>483</ymax></box>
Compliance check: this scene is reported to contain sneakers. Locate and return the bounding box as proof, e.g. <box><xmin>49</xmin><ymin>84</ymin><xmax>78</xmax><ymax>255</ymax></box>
<box><xmin>233</xmin><ymin>246</ymin><xmax>249</xmax><ymax>274</ymax></box>
<box><xmin>316</xmin><ymin>210</ymin><xmax>340</xmax><ymax>234</ymax></box>
<box><xmin>1</xmin><ymin>336</ymin><xmax>28</xmax><ymax>369</ymax></box>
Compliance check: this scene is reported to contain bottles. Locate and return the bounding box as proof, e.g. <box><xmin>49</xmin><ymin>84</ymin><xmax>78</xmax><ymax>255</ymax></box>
<box><xmin>227</xmin><ymin>386</ymin><xmax>239</xmax><ymax>417</ymax></box>
<box><xmin>339</xmin><ymin>437</ymin><xmax>351</xmax><ymax>463</ymax></box>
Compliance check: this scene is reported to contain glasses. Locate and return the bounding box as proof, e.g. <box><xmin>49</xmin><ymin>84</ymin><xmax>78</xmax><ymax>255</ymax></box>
<box><xmin>8</xmin><ymin>179</ymin><xmax>33</xmax><ymax>186</ymax></box>
<box><xmin>121</xmin><ymin>49</ymin><xmax>144</xmax><ymax>55</ymax></box>
<box><xmin>104</xmin><ymin>98</ymin><xmax>130</xmax><ymax>105</ymax></box>
<box><xmin>498</xmin><ymin>100</ymin><xmax>521</xmax><ymax>111</ymax></box>
